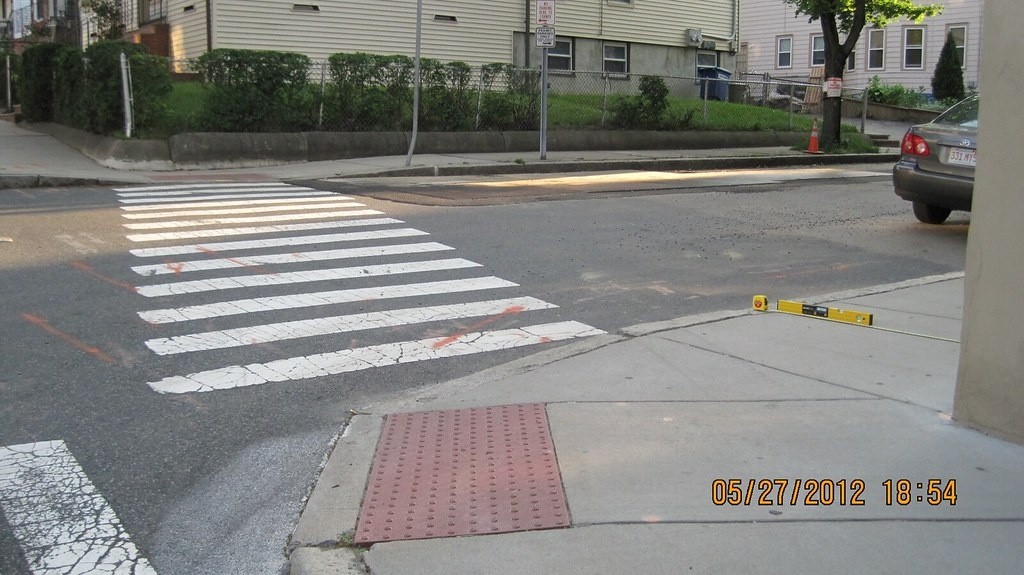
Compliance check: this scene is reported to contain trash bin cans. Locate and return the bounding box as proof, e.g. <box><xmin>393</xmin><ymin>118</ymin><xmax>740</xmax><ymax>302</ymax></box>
<box><xmin>697</xmin><ymin>65</ymin><xmax>733</xmax><ymax>101</ymax></box>
<box><xmin>728</xmin><ymin>83</ymin><xmax>748</xmax><ymax>104</ymax></box>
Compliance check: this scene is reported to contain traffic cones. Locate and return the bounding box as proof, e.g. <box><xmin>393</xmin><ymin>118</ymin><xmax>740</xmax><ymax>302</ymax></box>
<box><xmin>802</xmin><ymin>119</ymin><xmax>827</xmax><ymax>154</ymax></box>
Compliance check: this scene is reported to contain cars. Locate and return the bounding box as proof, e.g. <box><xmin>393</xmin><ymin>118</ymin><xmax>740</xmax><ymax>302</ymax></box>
<box><xmin>892</xmin><ymin>93</ymin><xmax>979</xmax><ymax>225</ymax></box>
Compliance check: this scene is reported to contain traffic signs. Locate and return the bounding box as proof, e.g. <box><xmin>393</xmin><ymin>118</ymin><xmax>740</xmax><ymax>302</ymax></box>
<box><xmin>535</xmin><ymin>0</ymin><xmax>555</xmax><ymax>25</ymax></box>
<box><xmin>535</xmin><ymin>27</ymin><xmax>556</xmax><ymax>48</ymax></box>
